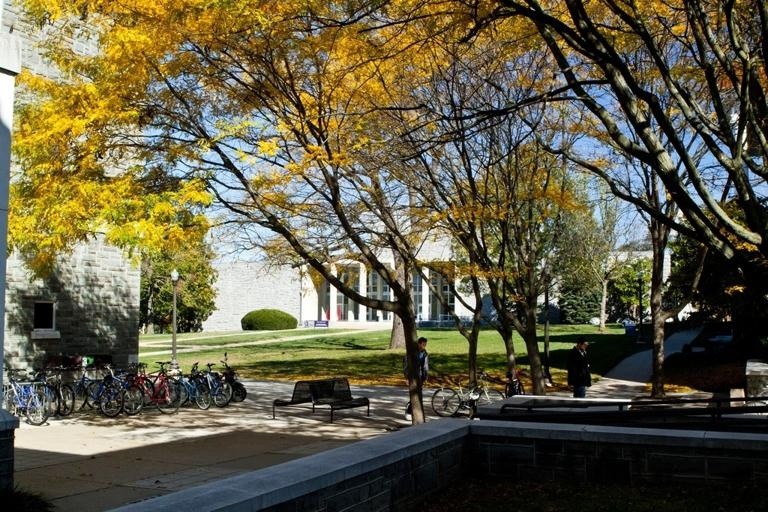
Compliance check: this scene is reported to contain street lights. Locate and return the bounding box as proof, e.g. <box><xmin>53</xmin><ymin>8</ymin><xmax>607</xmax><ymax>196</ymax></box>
<box><xmin>170</xmin><ymin>270</ymin><xmax>180</xmax><ymax>372</ymax></box>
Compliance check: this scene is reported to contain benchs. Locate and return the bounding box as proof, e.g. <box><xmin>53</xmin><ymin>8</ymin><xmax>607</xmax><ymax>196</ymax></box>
<box><xmin>308</xmin><ymin>378</ymin><xmax>369</xmax><ymax>423</ymax></box>
<box><xmin>273</xmin><ymin>381</ymin><xmax>334</xmax><ymax>419</ymax></box>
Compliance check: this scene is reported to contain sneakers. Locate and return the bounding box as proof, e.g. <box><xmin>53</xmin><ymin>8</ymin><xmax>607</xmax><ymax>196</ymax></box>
<box><xmin>405</xmin><ymin>413</ymin><xmax>412</xmax><ymax>421</ymax></box>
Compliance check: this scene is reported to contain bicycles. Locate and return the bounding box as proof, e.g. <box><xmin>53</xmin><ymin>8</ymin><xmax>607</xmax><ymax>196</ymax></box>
<box><xmin>1</xmin><ymin>352</ymin><xmax>246</xmax><ymax>427</ymax></box>
<box><xmin>431</xmin><ymin>367</ymin><xmax>506</xmax><ymax>417</ymax></box>
<box><xmin>503</xmin><ymin>369</ymin><xmax>529</xmax><ymax>398</ymax></box>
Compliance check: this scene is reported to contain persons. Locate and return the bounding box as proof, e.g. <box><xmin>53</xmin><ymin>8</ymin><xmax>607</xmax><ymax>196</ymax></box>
<box><xmin>56</xmin><ymin>350</ymin><xmax>94</xmax><ymax>368</ymax></box>
<box><xmin>402</xmin><ymin>336</ymin><xmax>429</xmax><ymax>421</ymax></box>
<box><xmin>615</xmin><ymin>309</ymin><xmax>653</xmax><ymax>334</ymax></box>
<box><xmin>567</xmin><ymin>339</ymin><xmax>592</xmax><ymax>398</ymax></box>
<box><xmin>682</xmin><ymin>311</ymin><xmax>693</xmax><ymax>320</ymax></box>
